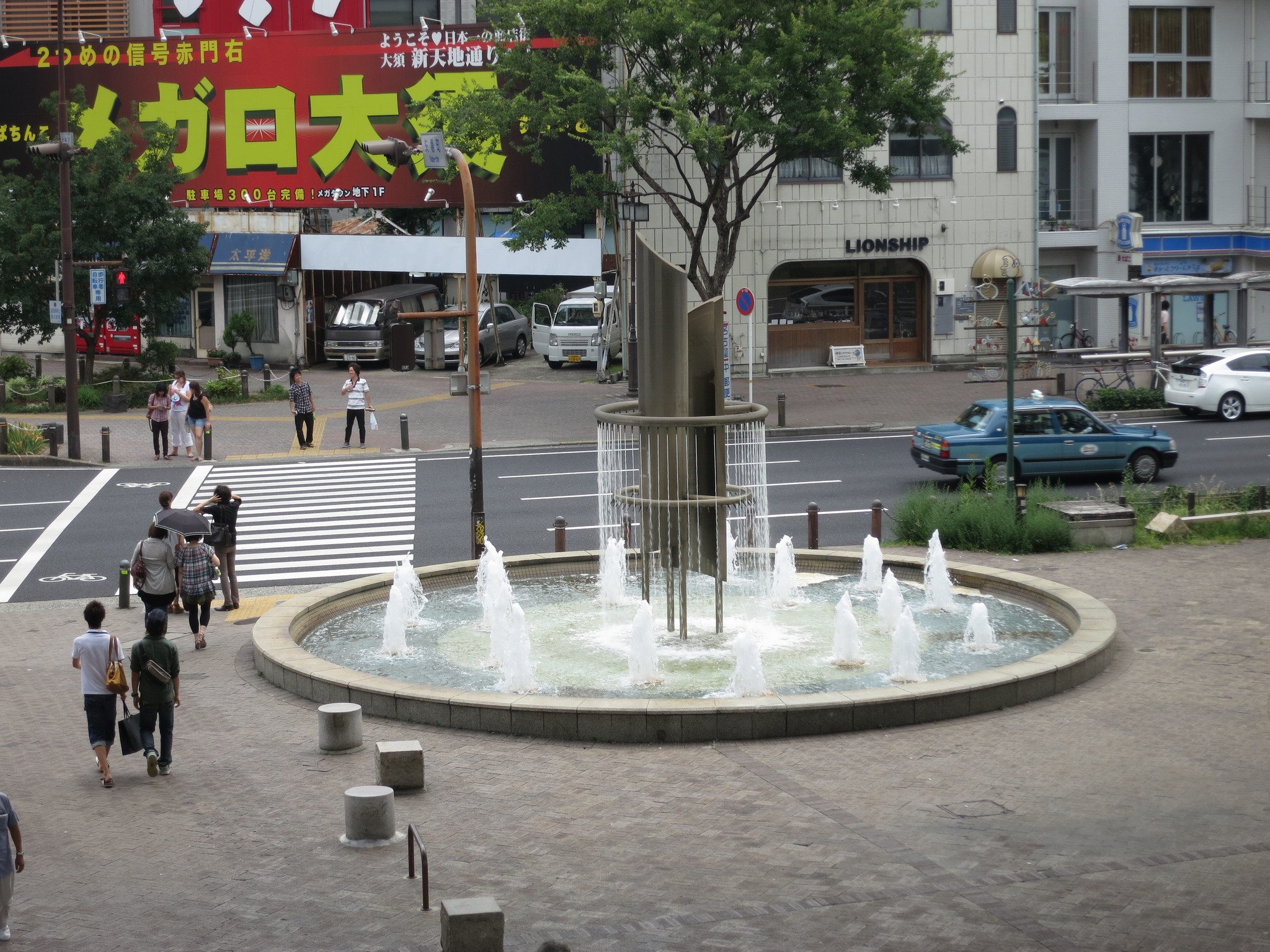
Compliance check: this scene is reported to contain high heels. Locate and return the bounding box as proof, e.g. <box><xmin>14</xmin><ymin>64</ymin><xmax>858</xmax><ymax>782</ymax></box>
<box><xmin>173</xmin><ymin>604</ymin><xmax>184</xmax><ymax>614</ymax></box>
<box><xmin>199</xmin><ymin>632</ymin><xmax>207</xmax><ymax>648</ymax></box>
<box><xmin>168</xmin><ymin>604</ymin><xmax>173</xmax><ymax>614</ymax></box>
<box><xmin>195</xmin><ymin>640</ymin><xmax>201</xmax><ymax>650</ymax></box>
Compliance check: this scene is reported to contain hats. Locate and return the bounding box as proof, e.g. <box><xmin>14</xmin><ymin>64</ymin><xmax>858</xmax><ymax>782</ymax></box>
<box><xmin>147</xmin><ymin>608</ymin><xmax>167</xmax><ymax>630</ymax></box>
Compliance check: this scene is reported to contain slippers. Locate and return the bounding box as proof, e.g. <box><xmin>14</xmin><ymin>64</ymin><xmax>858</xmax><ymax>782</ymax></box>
<box><xmin>189</xmin><ymin>453</ymin><xmax>194</xmax><ymax>458</ymax></box>
<box><xmin>168</xmin><ymin>453</ymin><xmax>177</xmax><ymax>456</ymax></box>
<box><xmin>198</xmin><ymin>457</ymin><xmax>203</xmax><ymax>461</ymax></box>
<box><xmin>191</xmin><ymin>455</ymin><xmax>198</xmax><ymax>461</ymax></box>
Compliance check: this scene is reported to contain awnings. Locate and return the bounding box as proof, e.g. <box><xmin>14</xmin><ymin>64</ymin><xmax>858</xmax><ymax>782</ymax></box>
<box><xmin>136</xmin><ymin>233</ymin><xmax>299</xmax><ymax>275</ymax></box>
<box><xmin>971</xmin><ymin>249</ymin><xmax>1023</xmax><ymax>278</ymax></box>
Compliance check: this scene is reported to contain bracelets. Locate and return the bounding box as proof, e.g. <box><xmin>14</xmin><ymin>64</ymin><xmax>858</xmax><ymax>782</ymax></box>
<box><xmin>207</xmin><ymin>421</ymin><xmax>210</xmax><ymax>423</ymax></box>
<box><xmin>179</xmin><ymin>587</ymin><xmax>182</xmax><ymax>590</ymax></box>
<box><xmin>1166</xmin><ymin>334</ymin><xmax>1169</xmax><ymax>336</ymax></box>
<box><xmin>174</xmin><ymin>551</ymin><xmax>176</xmax><ymax>554</ymax></box>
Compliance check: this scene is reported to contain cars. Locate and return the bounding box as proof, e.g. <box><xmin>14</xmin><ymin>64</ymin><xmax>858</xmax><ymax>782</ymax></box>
<box><xmin>785</xmin><ymin>284</ymin><xmax>888</xmax><ymax>323</ymax></box>
<box><xmin>414</xmin><ymin>303</ymin><xmax>530</xmax><ymax>371</ymax></box>
<box><xmin>1163</xmin><ymin>347</ymin><xmax>1270</xmax><ymax>422</ymax></box>
<box><xmin>910</xmin><ymin>389</ymin><xmax>1180</xmax><ymax>493</ymax></box>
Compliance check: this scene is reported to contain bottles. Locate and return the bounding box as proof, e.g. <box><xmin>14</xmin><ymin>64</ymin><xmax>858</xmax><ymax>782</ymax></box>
<box><xmin>313</xmin><ymin>411</ymin><xmax>316</xmax><ymax>420</ymax></box>
<box><xmin>174</xmin><ymin>700</ymin><xmax>178</xmax><ymax>707</ymax></box>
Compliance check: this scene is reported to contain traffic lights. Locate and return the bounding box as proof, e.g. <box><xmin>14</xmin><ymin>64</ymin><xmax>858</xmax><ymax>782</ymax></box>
<box><xmin>28</xmin><ymin>141</ymin><xmax>73</xmax><ymax>162</ymax></box>
<box><xmin>361</xmin><ymin>136</ymin><xmax>412</xmax><ymax>169</ymax></box>
<box><xmin>112</xmin><ymin>268</ymin><xmax>133</xmax><ymax>304</ymax></box>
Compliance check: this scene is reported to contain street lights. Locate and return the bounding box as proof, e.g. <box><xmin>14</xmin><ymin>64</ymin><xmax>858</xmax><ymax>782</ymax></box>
<box><xmin>621</xmin><ymin>180</ymin><xmax>650</xmax><ymax>398</ymax></box>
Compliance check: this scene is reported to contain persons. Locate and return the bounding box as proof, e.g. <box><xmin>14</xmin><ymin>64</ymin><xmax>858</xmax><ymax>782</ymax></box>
<box><xmin>131</xmin><ymin>521</ymin><xmax>179</xmax><ymax>637</ymax></box>
<box><xmin>289</xmin><ymin>368</ymin><xmax>316</xmax><ymax>449</ymax></box>
<box><xmin>71</xmin><ymin>599</ymin><xmax>127</xmax><ymax>787</ymax></box>
<box><xmin>153</xmin><ymin>492</ymin><xmax>184</xmax><ymax>614</ymax></box>
<box><xmin>147</xmin><ymin>370</ymin><xmax>210</xmax><ymax>461</ymax></box>
<box><xmin>0</xmin><ymin>791</ymin><xmax>25</xmax><ymax>940</ymax></box>
<box><xmin>1161</xmin><ymin>300</ymin><xmax>1170</xmax><ymax>344</ymax></box>
<box><xmin>130</xmin><ymin>609</ymin><xmax>181</xmax><ymax>777</ymax></box>
<box><xmin>1057</xmin><ymin>413</ymin><xmax>1093</xmax><ymax>434</ymax></box>
<box><xmin>193</xmin><ymin>485</ymin><xmax>242</xmax><ymax>611</ymax></box>
<box><xmin>341</xmin><ymin>365</ymin><xmax>373</xmax><ymax>448</ymax></box>
<box><xmin>174</xmin><ymin>535</ymin><xmax>220</xmax><ymax>650</ymax></box>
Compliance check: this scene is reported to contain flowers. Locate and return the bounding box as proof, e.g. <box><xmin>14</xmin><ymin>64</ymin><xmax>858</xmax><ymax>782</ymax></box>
<box><xmin>206</xmin><ymin>347</ymin><xmax>226</xmax><ymax>358</ymax></box>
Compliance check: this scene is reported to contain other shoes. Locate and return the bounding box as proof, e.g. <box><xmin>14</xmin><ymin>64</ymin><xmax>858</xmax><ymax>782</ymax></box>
<box><xmin>342</xmin><ymin>442</ymin><xmax>350</xmax><ymax>447</ymax></box>
<box><xmin>360</xmin><ymin>443</ymin><xmax>366</xmax><ymax>448</ymax></box>
<box><xmin>214</xmin><ymin>604</ymin><xmax>233</xmax><ymax>611</ymax></box>
<box><xmin>233</xmin><ymin>603</ymin><xmax>239</xmax><ymax>609</ymax></box>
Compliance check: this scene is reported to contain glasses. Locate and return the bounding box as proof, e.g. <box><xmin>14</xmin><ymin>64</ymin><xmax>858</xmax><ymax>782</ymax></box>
<box><xmin>176</xmin><ymin>376</ymin><xmax>182</xmax><ymax>380</ymax></box>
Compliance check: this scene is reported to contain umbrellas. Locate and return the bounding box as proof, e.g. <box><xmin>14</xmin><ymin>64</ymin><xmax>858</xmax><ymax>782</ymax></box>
<box><xmin>154</xmin><ymin>509</ymin><xmax>211</xmax><ymax>537</ymax></box>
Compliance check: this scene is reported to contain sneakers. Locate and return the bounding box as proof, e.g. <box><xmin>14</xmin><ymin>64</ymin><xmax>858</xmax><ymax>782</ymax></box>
<box><xmin>95</xmin><ymin>755</ymin><xmax>170</xmax><ymax>788</ymax></box>
<box><xmin>0</xmin><ymin>924</ymin><xmax>10</xmax><ymax>940</ymax></box>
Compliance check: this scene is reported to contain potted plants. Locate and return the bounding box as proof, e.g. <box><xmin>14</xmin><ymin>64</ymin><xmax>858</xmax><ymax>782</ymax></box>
<box><xmin>1044</xmin><ymin>215</ymin><xmax>1073</xmax><ymax>231</ymax></box>
<box><xmin>222</xmin><ymin>312</ymin><xmax>264</xmax><ymax>371</ymax></box>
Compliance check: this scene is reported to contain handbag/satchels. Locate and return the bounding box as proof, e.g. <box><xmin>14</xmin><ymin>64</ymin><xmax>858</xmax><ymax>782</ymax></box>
<box><xmin>130</xmin><ymin>540</ymin><xmax>146</xmax><ymax>579</ymax></box>
<box><xmin>370</xmin><ymin>411</ymin><xmax>378</xmax><ymax>431</ymax></box>
<box><xmin>146</xmin><ymin>393</ymin><xmax>156</xmax><ymax>419</ymax></box>
<box><xmin>203</xmin><ymin>504</ymin><xmax>229</xmax><ymax>547</ymax></box>
<box><xmin>105</xmin><ymin>635</ymin><xmax>172</xmax><ymax>756</ymax></box>
<box><xmin>208</xmin><ymin>400</ymin><xmax>212</xmax><ymax>411</ymax></box>
<box><xmin>205</xmin><ymin>544</ymin><xmax>219</xmax><ymax>580</ymax></box>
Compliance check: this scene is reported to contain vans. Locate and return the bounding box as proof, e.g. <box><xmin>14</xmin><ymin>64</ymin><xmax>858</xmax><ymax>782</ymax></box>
<box><xmin>76</xmin><ymin>313</ymin><xmax>141</xmax><ymax>356</ymax></box>
<box><xmin>323</xmin><ymin>284</ymin><xmax>446</xmax><ymax>366</ymax></box>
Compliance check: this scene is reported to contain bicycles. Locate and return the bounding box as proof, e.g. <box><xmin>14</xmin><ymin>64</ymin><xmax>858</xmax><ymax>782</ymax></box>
<box><xmin>1075</xmin><ymin>356</ymin><xmax>1136</xmax><ymax>408</ymax></box>
<box><xmin>1145</xmin><ymin>359</ymin><xmax>1170</xmax><ymax>390</ymax></box>
<box><xmin>1213</xmin><ymin>312</ymin><xmax>1237</xmax><ymax>344</ymax></box>
<box><xmin>1060</xmin><ymin>320</ymin><xmax>1096</xmax><ymax>364</ymax></box>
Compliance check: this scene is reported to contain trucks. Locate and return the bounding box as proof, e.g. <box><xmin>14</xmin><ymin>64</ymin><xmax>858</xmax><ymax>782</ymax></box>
<box><xmin>531</xmin><ymin>284</ymin><xmax>622</xmax><ymax>369</ymax></box>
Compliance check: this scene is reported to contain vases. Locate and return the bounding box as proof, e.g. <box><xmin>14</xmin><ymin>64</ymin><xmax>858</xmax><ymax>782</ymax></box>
<box><xmin>206</xmin><ymin>357</ymin><xmax>224</xmax><ymax>367</ymax></box>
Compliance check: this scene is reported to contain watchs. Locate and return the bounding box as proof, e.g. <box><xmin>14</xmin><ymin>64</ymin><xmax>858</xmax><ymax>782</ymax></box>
<box><xmin>16</xmin><ymin>851</ymin><xmax>24</xmax><ymax>857</ymax></box>
<box><xmin>131</xmin><ymin>692</ymin><xmax>138</xmax><ymax>697</ymax></box>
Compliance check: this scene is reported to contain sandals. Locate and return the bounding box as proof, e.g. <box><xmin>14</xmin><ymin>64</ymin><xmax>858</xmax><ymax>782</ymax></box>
<box><xmin>306</xmin><ymin>442</ymin><xmax>313</xmax><ymax>447</ymax></box>
<box><xmin>300</xmin><ymin>445</ymin><xmax>306</xmax><ymax>449</ymax></box>
<box><xmin>154</xmin><ymin>455</ymin><xmax>160</xmax><ymax>460</ymax></box>
<box><xmin>164</xmin><ymin>456</ymin><xmax>172</xmax><ymax>460</ymax></box>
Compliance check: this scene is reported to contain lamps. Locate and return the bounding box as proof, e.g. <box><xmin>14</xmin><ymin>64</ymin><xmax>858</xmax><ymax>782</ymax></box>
<box><xmin>424</xmin><ymin>188</ymin><xmax>450</xmax><ymax>208</ymax></box>
<box><xmin>516</xmin><ymin>194</ymin><xmax>529</xmax><ymax>202</ymax></box>
<box><xmin>776</xmin><ymin>201</ymin><xmax>782</xmax><ymax>210</ymax></box>
<box><xmin>832</xmin><ymin>200</ymin><xmax>839</xmax><ymax>210</ymax></box>
<box><xmin>420</xmin><ymin>17</ymin><xmax>445</xmax><ymax>32</ymax></box>
<box><xmin>165</xmin><ymin>196</ymin><xmax>189</xmax><ymax>208</ymax></box>
<box><xmin>330</xmin><ymin>22</ymin><xmax>354</xmax><ymax>36</ymax></box>
<box><xmin>159</xmin><ymin>28</ymin><xmax>184</xmax><ymax>42</ymax></box>
<box><xmin>243</xmin><ymin>26</ymin><xmax>267</xmax><ymax>40</ymax></box>
<box><xmin>893</xmin><ymin>199</ymin><xmax>899</xmax><ymax>208</ymax></box>
<box><xmin>333</xmin><ymin>188</ymin><xmax>357</xmax><ymax>208</ymax></box>
<box><xmin>244</xmin><ymin>194</ymin><xmax>273</xmax><ymax>208</ymax></box>
<box><xmin>950</xmin><ymin>195</ymin><xmax>957</xmax><ymax>206</ymax></box>
<box><xmin>1</xmin><ymin>35</ymin><xmax>26</xmax><ymax>48</ymax></box>
<box><xmin>77</xmin><ymin>30</ymin><xmax>103</xmax><ymax>43</ymax></box>
